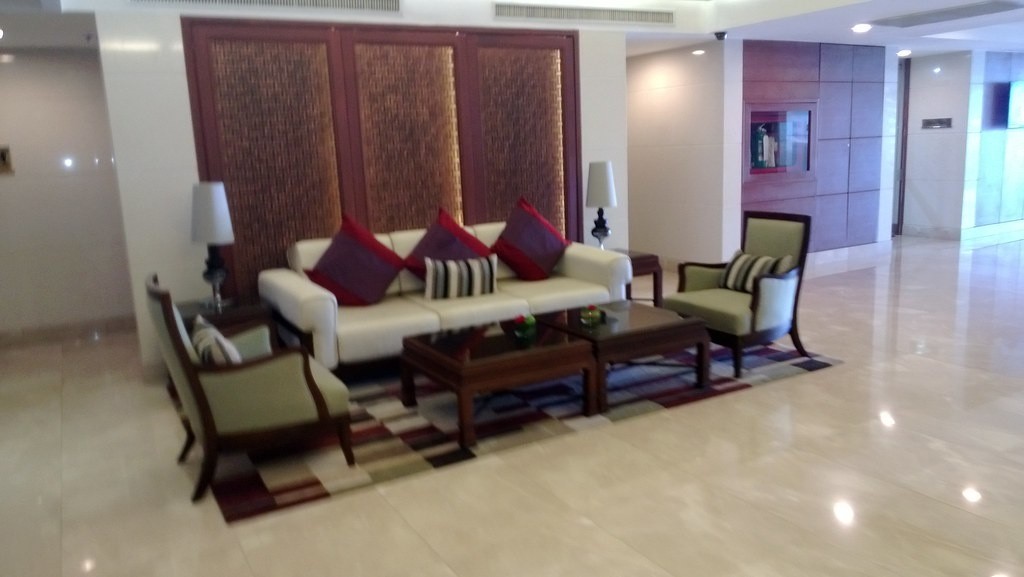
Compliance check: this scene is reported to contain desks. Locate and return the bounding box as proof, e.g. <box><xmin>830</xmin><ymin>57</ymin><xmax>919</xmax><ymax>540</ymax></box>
<box><xmin>180</xmin><ymin>301</ymin><xmax>266</xmax><ymax>330</ymax></box>
<box><xmin>625</xmin><ymin>249</ymin><xmax>663</xmax><ymax>309</ymax></box>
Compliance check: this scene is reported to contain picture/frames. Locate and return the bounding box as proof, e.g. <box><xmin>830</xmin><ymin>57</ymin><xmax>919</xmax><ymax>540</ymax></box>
<box><xmin>741</xmin><ymin>100</ymin><xmax>820</xmax><ymax>185</ymax></box>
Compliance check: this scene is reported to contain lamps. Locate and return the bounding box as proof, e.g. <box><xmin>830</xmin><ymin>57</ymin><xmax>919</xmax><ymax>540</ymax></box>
<box><xmin>584</xmin><ymin>161</ymin><xmax>617</xmax><ymax>249</ymax></box>
<box><xmin>186</xmin><ymin>179</ymin><xmax>236</xmax><ymax>312</ymax></box>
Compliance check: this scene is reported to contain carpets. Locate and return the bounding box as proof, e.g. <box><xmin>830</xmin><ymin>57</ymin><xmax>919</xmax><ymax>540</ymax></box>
<box><xmin>207</xmin><ymin>337</ymin><xmax>844</xmax><ymax>524</ymax></box>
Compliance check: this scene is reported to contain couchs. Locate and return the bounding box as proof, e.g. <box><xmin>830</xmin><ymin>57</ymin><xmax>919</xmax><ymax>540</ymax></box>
<box><xmin>259</xmin><ymin>220</ymin><xmax>632</xmax><ymax>386</ymax></box>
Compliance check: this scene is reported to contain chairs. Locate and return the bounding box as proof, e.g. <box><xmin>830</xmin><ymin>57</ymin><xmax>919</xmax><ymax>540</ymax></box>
<box><xmin>144</xmin><ymin>272</ymin><xmax>357</xmax><ymax>502</ymax></box>
<box><xmin>664</xmin><ymin>211</ymin><xmax>812</xmax><ymax>378</ymax></box>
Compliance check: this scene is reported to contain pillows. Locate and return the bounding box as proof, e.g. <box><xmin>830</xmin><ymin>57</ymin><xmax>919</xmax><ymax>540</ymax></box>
<box><xmin>490</xmin><ymin>196</ymin><xmax>572</xmax><ymax>280</ymax></box>
<box><xmin>304</xmin><ymin>218</ymin><xmax>405</xmax><ymax>304</ymax></box>
<box><xmin>425</xmin><ymin>252</ymin><xmax>499</xmax><ymax>299</ymax></box>
<box><xmin>193</xmin><ymin>314</ymin><xmax>242</xmax><ymax>368</ymax></box>
<box><xmin>403</xmin><ymin>208</ymin><xmax>492</xmax><ymax>281</ymax></box>
<box><xmin>720</xmin><ymin>249</ymin><xmax>793</xmax><ymax>294</ymax></box>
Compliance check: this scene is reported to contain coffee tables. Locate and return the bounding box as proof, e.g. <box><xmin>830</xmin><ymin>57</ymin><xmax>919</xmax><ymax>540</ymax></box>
<box><xmin>538</xmin><ymin>298</ymin><xmax>712</xmax><ymax>413</ymax></box>
<box><xmin>401</xmin><ymin>319</ymin><xmax>601</xmax><ymax>450</ymax></box>
